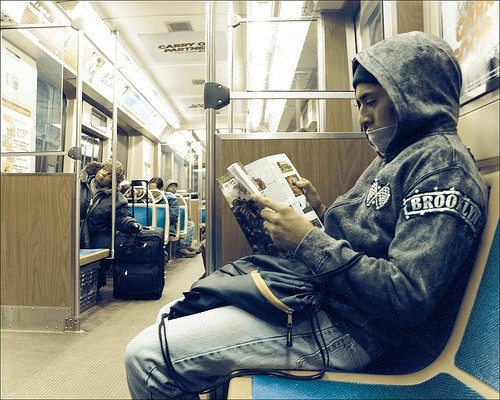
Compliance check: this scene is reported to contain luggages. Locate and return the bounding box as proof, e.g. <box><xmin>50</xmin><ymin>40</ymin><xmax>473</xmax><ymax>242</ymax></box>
<box><xmin>114</xmin><ymin>180</ymin><xmax>164</xmax><ymax>300</ymax></box>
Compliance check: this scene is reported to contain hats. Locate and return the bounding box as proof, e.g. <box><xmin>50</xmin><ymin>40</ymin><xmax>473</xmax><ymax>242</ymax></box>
<box><xmin>85</xmin><ymin>160</ymin><xmax>124</xmax><ymax>183</ymax></box>
<box><xmin>165</xmin><ymin>180</ymin><xmax>178</xmax><ymax>189</ymax></box>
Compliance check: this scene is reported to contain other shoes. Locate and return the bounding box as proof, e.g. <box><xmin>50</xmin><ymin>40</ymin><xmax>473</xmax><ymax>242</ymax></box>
<box><xmin>176</xmin><ymin>248</ymin><xmax>199</xmax><ymax>257</ymax></box>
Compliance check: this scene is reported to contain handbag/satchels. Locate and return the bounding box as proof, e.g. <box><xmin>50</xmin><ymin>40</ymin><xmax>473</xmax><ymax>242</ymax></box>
<box><xmin>79</xmin><ymin>218</ymin><xmax>90</xmax><ymax>249</ymax></box>
<box><xmin>155</xmin><ymin>252</ymin><xmax>368</xmax><ymax>394</ymax></box>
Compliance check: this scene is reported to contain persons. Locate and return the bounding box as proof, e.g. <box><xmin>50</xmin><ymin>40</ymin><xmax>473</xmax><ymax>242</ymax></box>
<box><xmin>81</xmin><ymin>160</ymin><xmax>143</xmax><ymax>298</ymax></box>
<box><xmin>124</xmin><ymin>32</ymin><xmax>488</xmax><ymax>400</ymax></box>
<box><xmin>120</xmin><ymin>178</ymin><xmax>202</xmax><ymax>258</ymax></box>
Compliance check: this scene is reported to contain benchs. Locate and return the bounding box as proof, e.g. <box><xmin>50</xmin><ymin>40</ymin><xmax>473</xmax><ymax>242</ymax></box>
<box><xmin>227</xmin><ymin>171</ymin><xmax>499</xmax><ymax>399</ymax></box>
<box><xmin>80</xmin><ymin>186</ymin><xmax>208</xmax><ymax>313</ymax></box>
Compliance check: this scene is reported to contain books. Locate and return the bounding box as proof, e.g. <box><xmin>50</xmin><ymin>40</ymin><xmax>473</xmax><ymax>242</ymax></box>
<box><xmin>216</xmin><ymin>153</ymin><xmax>325</xmax><ymax>259</ymax></box>
